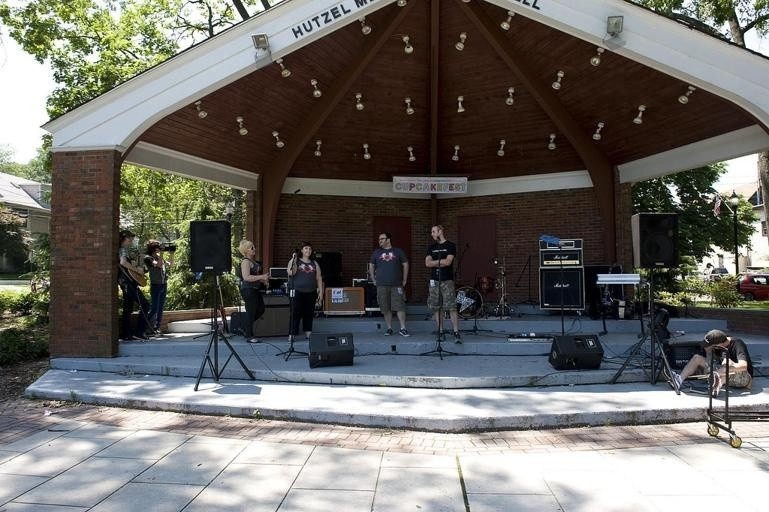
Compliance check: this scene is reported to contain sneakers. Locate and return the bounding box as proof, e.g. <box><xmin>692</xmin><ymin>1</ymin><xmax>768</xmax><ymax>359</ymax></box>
<box><xmin>708</xmin><ymin>371</ymin><xmax>721</xmax><ymax>398</ymax></box>
<box><xmin>436</xmin><ymin>329</ymin><xmax>446</xmax><ymax>342</ymax></box>
<box><xmin>399</xmin><ymin>327</ymin><xmax>411</xmax><ymax>337</ymax></box>
<box><xmin>453</xmin><ymin>330</ymin><xmax>462</xmax><ymax>344</ymax></box>
<box><xmin>384</xmin><ymin>328</ymin><xmax>393</xmax><ymax>336</ymax></box>
<box><xmin>117</xmin><ymin>328</ymin><xmax>164</xmax><ymax>343</ymax></box>
<box><xmin>287</xmin><ymin>332</ymin><xmax>311</xmax><ymax>343</ymax></box>
<box><xmin>663</xmin><ymin>365</ymin><xmax>682</xmax><ymax>390</ymax></box>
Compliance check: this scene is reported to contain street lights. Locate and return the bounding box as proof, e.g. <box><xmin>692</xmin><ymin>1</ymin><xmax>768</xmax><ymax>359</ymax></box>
<box><xmin>729</xmin><ymin>189</ymin><xmax>740</xmax><ymax>280</ymax></box>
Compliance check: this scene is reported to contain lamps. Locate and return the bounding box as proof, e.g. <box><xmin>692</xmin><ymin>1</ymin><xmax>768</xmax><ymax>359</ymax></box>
<box><xmin>593</xmin><ymin>122</ymin><xmax>604</xmax><ymax>140</ymax></box>
<box><xmin>607</xmin><ymin>15</ymin><xmax>623</xmax><ymax>33</ymax></box>
<box><xmin>236</xmin><ymin>116</ymin><xmax>249</xmax><ymax>136</ymax></box>
<box><xmin>272</xmin><ymin>130</ymin><xmax>285</xmax><ymax>149</ymax></box>
<box><xmin>405</xmin><ymin>99</ymin><xmax>414</xmax><ymax>115</ymax></box>
<box><xmin>251</xmin><ymin>34</ymin><xmax>270</xmax><ymax>51</ymax></box>
<box><xmin>194</xmin><ymin>100</ymin><xmax>207</xmax><ymax>120</ymax></box>
<box><xmin>551</xmin><ymin>70</ymin><xmax>564</xmax><ymax>89</ymax></box>
<box><xmin>357</xmin><ymin>14</ymin><xmax>371</xmax><ymax>36</ymax></box>
<box><xmin>590</xmin><ymin>46</ymin><xmax>604</xmax><ymax>66</ymax></box>
<box><xmin>548</xmin><ymin>134</ymin><xmax>556</xmax><ymax>150</ymax></box>
<box><xmin>452</xmin><ymin>146</ymin><xmax>460</xmax><ymax>162</ymax></box>
<box><xmin>679</xmin><ymin>86</ymin><xmax>696</xmax><ymax>104</ymax></box>
<box><xmin>355</xmin><ymin>93</ymin><xmax>364</xmax><ymax>112</ymax></box>
<box><xmin>363</xmin><ymin>143</ymin><xmax>370</xmax><ymax>160</ymax></box>
<box><xmin>455</xmin><ymin>32</ymin><xmax>466</xmax><ymax>51</ymax></box>
<box><xmin>497</xmin><ymin>140</ymin><xmax>506</xmax><ymax>157</ymax></box>
<box><xmin>313</xmin><ymin>140</ymin><xmax>323</xmax><ymax>157</ymax></box>
<box><xmin>408</xmin><ymin>147</ymin><xmax>415</xmax><ymax>162</ymax></box>
<box><xmin>457</xmin><ymin>96</ymin><xmax>465</xmax><ymax>113</ymax></box>
<box><xmin>505</xmin><ymin>86</ymin><xmax>515</xmax><ymax>105</ymax></box>
<box><xmin>402</xmin><ymin>35</ymin><xmax>414</xmax><ymax>55</ymax></box>
<box><xmin>634</xmin><ymin>105</ymin><xmax>646</xmax><ymax>124</ymax></box>
<box><xmin>395</xmin><ymin>0</ymin><xmax>407</xmax><ymax>7</ymax></box>
<box><xmin>275</xmin><ymin>58</ymin><xmax>291</xmax><ymax>79</ymax></box>
<box><xmin>500</xmin><ymin>11</ymin><xmax>514</xmax><ymax>30</ymax></box>
<box><xmin>310</xmin><ymin>79</ymin><xmax>322</xmax><ymax>99</ymax></box>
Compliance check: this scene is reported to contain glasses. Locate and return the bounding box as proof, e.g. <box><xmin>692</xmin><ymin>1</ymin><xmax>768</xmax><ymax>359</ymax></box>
<box><xmin>247</xmin><ymin>246</ymin><xmax>254</xmax><ymax>251</ymax></box>
<box><xmin>378</xmin><ymin>237</ymin><xmax>387</xmax><ymax>241</ymax></box>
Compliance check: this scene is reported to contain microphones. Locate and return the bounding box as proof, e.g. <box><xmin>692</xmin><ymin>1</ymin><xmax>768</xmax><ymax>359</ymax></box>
<box><xmin>293</xmin><ymin>252</ymin><xmax>297</xmax><ymax>259</ymax></box>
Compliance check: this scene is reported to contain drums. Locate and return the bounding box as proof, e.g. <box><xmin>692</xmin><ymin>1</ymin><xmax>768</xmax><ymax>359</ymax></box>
<box><xmin>455</xmin><ymin>286</ymin><xmax>484</xmax><ymax>318</ymax></box>
<box><xmin>478</xmin><ymin>276</ymin><xmax>493</xmax><ymax>294</ymax></box>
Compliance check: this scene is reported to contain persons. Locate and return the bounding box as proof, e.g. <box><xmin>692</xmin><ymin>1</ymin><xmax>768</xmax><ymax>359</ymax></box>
<box><xmin>143</xmin><ymin>238</ymin><xmax>176</xmax><ymax>334</ymax></box>
<box><xmin>369</xmin><ymin>233</ymin><xmax>411</xmax><ymax>337</ymax></box>
<box><xmin>117</xmin><ymin>229</ymin><xmax>151</xmax><ymax>343</ymax></box>
<box><xmin>238</xmin><ymin>240</ymin><xmax>270</xmax><ymax>344</ymax></box>
<box><xmin>424</xmin><ymin>224</ymin><xmax>463</xmax><ymax>344</ymax></box>
<box><xmin>286</xmin><ymin>240</ymin><xmax>323</xmax><ymax>342</ymax></box>
<box><xmin>661</xmin><ymin>329</ymin><xmax>754</xmax><ymax>398</ymax></box>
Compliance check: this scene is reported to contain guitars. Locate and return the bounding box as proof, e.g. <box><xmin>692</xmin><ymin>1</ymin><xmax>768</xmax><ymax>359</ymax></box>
<box><xmin>128</xmin><ymin>248</ymin><xmax>147</xmax><ymax>286</ymax></box>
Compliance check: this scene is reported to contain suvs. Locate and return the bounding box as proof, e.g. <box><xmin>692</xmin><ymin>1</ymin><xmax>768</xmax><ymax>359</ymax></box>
<box><xmin>699</xmin><ymin>266</ymin><xmax>730</xmax><ymax>283</ymax></box>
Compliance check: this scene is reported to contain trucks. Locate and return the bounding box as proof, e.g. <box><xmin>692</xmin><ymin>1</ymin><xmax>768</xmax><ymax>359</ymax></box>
<box><xmin>672</xmin><ymin>256</ymin><xmax>708</xmax><ymax>286</ymax></box>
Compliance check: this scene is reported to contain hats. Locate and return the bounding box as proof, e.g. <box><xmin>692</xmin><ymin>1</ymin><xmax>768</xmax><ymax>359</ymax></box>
<box><xmin>699</xmin><ymin>329</ymin><xmax>727</xmax><ymax>349</ymax></box>
<box><xmin>119</xmin><ymin>229</ymin><xmax>136</xmax><ymax>241</ymax></box>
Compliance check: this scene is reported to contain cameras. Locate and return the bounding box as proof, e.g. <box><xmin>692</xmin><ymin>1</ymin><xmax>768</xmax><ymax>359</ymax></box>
<box><xmin>159</xmin><ymin>242</ymin><xmax>176</xmax><ymax>252</ymax></box>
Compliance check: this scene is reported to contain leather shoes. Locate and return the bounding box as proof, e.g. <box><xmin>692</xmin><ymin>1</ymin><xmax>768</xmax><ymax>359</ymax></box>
<box><xmin>237</xmin><ymin>327</ymin><xmax>262</xmax><ymax>343</ymax></box>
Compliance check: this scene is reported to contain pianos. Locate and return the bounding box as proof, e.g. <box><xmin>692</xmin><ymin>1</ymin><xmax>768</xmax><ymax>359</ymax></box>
<box><xmin>596</xmin><ymin>273</ymin><xmax>647</xmax><ymax>285</ymax></box>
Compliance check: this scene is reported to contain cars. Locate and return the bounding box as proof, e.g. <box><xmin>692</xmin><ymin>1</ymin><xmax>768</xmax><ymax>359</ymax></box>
<box><xmin>733</xmin><ymin>272</ymin><xmax>769</xmax><ymax>301</ymax></box>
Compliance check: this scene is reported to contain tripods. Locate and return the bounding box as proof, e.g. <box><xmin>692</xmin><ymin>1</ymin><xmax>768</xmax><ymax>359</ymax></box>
<box><xmin>420</xmin><ymin>247</ymin><xmax>458</xmax><ymax>360</ymax></box>
<box><xmin>490</xmin><ymin>257</ymin><xmax>522</xmax><ymax>318</ymax></box>
<box><xmin>192</xmin><ymin>281</ymin><xmax>229</xmax><ymax>339</ymax></box>
<box><xmin>194</xmin><ymin>274</ymin><xmax>255</xmax><ymax>392</ymax></box>
<box><xmin>275</xmin><ymin>259</ymin><xmax>308</xmax><ymax>362</ymax></box>
<box><xmin>459</xmin><ymin>317</ymin><xmax>492</xmax><ymax>334</ymax></box>
<box><xmin>611</xmin><ymin>270</ymin><xmax>680</xmax><ymax>396</ymax></box>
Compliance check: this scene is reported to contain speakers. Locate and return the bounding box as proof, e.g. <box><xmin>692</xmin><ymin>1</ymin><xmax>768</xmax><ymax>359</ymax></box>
<box><xmin>630</xmin><ymin>212</ymin><xmax>680</xmax><ymax>268</ymax></box>
<box><xmin>190</xmin><ymin>221</ymin><xmax>232</xmax><ymax>273</ymax></box>
<box><xmin>308</xmin><ymin>332</ymin><xmax>354</xmax><ymax>369</ymax></box>
<box><xmin>548</xmin><ymin>335</ymin><xmax>604</xmax><ymax>371</ymax></box>
<box><xmin>540</xmin><ymin>267</ymin><xmax>584</xmax><ymax>309</ymax></box>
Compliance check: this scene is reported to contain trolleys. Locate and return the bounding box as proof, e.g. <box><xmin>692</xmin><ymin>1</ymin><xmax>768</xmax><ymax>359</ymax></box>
<box><xmin>704</xmin><ymin>344</ymin><xmax>769</xmax><ymax>448</ymax></box>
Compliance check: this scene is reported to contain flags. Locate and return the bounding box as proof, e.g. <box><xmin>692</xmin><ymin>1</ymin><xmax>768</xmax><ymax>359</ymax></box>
<box><xmin>712</xmin><ymin>195</ymin><xmax>722</xmax><ymax>218</ymax></box>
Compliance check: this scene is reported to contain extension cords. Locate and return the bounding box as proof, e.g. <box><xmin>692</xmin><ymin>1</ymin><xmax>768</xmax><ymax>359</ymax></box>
<box><xmin>506</xmin><ymin>336</ymin><xmax>555</xmax><ymax>343</ymax></box>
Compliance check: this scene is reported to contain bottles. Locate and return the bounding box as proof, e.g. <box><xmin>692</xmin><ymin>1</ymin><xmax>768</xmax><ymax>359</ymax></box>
<box><xmin>272</xmin><ymin>288</ymin><xmax>283</xmax><ymax>296</ymax></box>
